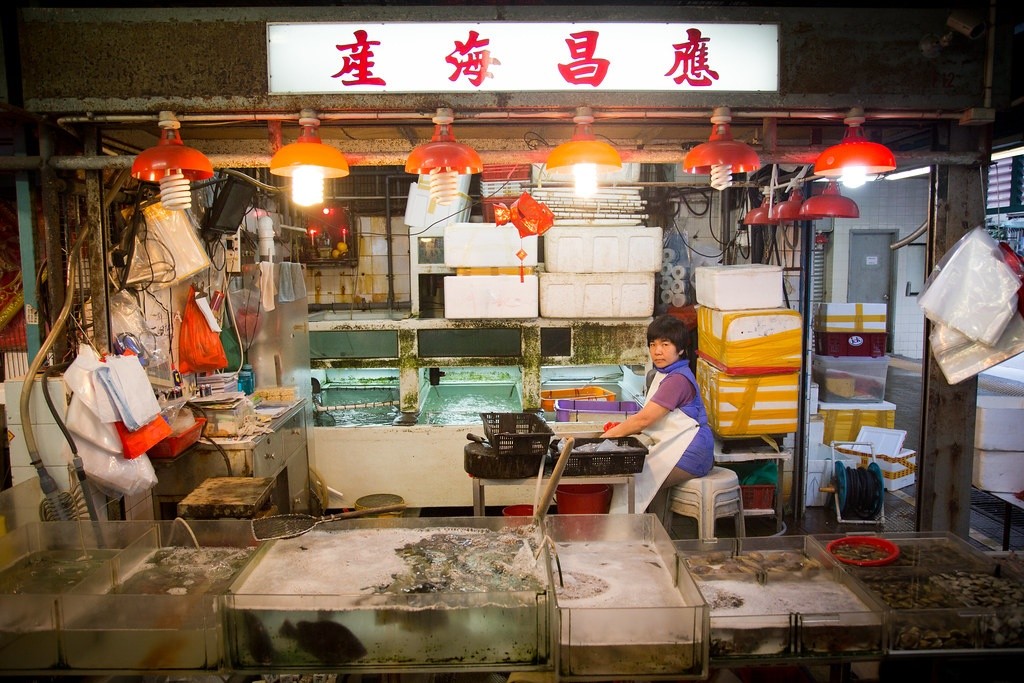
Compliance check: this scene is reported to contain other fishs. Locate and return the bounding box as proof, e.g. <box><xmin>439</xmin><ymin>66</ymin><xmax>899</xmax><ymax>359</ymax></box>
<box><xmin>279</xmin><ymin>619</ymin><xmax>368</xmax><ymax>665</ymax></box>
<box><xmin>243</xmin><ymin>613</ymin><xmax>274</xmax><ymax>663</ymax></box>
<box><xmin>374</xmin><ymin>609</ymin><xmax>450</xmax><ymax>633</ymax></box>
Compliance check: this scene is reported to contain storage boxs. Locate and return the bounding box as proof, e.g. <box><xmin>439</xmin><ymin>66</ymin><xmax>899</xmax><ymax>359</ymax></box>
<box><xmin>692</xmin><ymin>264</ymin><xmax>917</xmax><ymax>511</ymax></box>
<box><xmin>545</xmin><ymin>418</ymin><xmax>648</xmax><ymax>477</ymax></box>
<box><xmin>479</xmin><ymin>411</ymin><xmax>554</xmax><ymax>455</ymax></box>
<box><xmin>443</xmin><ymin>220</ymin><xmax>663</xmax><ymax>318</ymax></box>
<box><xmin>972</xmin><ymin>394</ymin><xmax>1024</xmax><ymax>453</ymax></box>
<box><xmin>972</xmin><ymin>448</ymin><xmax>1024</xmax><ymax>493</ymax></box>
<box><xmin>404</xmin><ymin>181</ymin><xmax>471</xmax><ymax>229</ymax></box>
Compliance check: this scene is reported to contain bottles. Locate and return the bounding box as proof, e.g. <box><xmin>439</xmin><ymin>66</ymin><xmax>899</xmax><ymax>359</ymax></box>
<box><xmin>238</xmin><ymin>364</ymin><xmax>255</xmax><ymax>395</ymax></box>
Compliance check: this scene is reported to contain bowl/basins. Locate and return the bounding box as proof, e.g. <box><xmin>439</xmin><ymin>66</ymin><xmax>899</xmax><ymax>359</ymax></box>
<box><xmin>827</xmin><ymin>536</ymin><xmax>900</xmax><ymax>566</ymax></box>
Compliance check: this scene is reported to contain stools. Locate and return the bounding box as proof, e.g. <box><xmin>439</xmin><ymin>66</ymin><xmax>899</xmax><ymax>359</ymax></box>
<box><xmin>658</xmin><ymin>465</ymin><xmax>746</xmax><ymax>541</ymax></box>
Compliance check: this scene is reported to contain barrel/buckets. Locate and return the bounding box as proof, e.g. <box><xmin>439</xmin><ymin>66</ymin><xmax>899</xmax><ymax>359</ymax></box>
<box><xmin>552</xmin><ymin>484</ymin><xmax>613</xmax><ymax>514</ymax></box>
<box><xmin>502</xmin><ymin>506</ymin><xmax>533</xmax><ymax>517</ymax></box>
<box><xmin>356</xmin><ymin>494</ymin><xmax>405</xmax><ymax>518</ymax></box>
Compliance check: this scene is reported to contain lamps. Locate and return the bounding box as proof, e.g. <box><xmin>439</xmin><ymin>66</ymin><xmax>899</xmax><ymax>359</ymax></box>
<box><xmin>270</xmin><ymin>108</ymin><xmax>350</xmax><ymax>208</ymax></box>
<box><xmin>404</xmin><ymin>116</ymin><xmax>483</xmax><ymax>204</ymax></box>
<box><xmin>682</xmin><ymin>105</ymin><xmax>760</xmax><ymax>192</ymax></box>
<box><xmin>813</xmin><ymin>107</ymin><xmax>897</xmax><ymax>188</ymax></box>
<box><xmin>741</xmin><ymin>175</ymin><xmax>859</xmax><ymax>226</ymax></box>
<box><xmin>546</xmin><ymin>108</ymin><xmax>621</xmax><ymax>196</ymax></box>
<box><xmin>131</xmin><ymin>109</ymin><xmax>214</xmax><ymax>211</ymax></box>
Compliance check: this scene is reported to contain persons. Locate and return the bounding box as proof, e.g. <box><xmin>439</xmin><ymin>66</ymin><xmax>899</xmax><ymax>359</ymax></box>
<box><xmin>599</xmin><ymin>314</ymin><xmax>714</xmax><ymax>516</ymax></box>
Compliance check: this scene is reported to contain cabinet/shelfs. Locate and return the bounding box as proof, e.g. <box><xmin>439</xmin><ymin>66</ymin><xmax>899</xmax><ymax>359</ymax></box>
<box><xmin>148</xmin><ymin>399</ymin><xmax>312</xmax><ymax>521</ymax></box>
